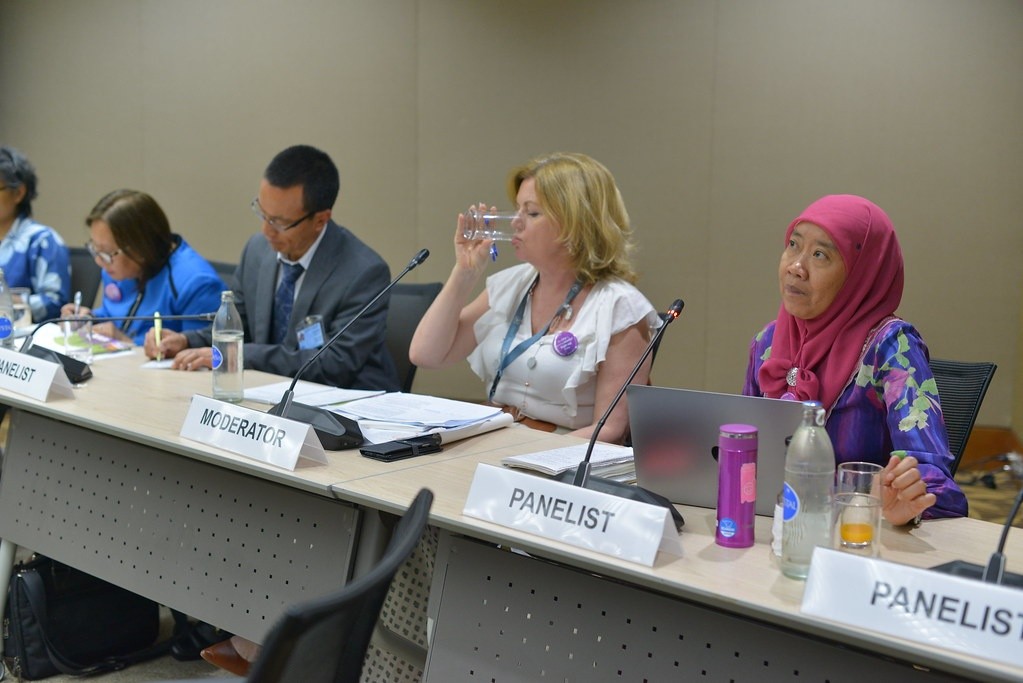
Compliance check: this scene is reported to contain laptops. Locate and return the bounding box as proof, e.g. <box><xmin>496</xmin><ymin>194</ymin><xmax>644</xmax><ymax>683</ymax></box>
<box><xmin>627</xmin><ymin>385</ymin><xmax>856</xmax><ymax>524</ymax></box>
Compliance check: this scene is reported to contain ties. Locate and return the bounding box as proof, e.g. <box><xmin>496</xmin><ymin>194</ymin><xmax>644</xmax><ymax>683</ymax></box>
<box><xmin>269</xmin><ymin>261</ymin><xmax>304</xmax><ymax>346</ymax></box>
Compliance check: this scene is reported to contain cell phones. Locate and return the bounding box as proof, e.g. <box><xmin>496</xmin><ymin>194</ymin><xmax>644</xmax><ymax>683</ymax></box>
<box><xmin>364</xmin><ymin>443</ymin><xmax>441</xmax><ymax>460</ymax></box>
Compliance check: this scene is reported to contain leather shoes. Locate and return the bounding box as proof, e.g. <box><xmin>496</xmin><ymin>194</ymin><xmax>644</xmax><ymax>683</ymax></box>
<box><xmin>171</xmin><ymin>622</ymin><xmax>234</xmax><ymax>661</ymax></box>
<box><xmin>199</xmin><ymin>638</ymin><xmax>259</xmax><ymax>677</ymax></box>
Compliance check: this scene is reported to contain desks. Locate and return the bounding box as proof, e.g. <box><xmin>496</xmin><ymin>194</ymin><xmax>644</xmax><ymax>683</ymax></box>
<box><xmin>0</xmin><ymin>325</ymin><xmax>1023</xmax><ymax>683</ymax></box>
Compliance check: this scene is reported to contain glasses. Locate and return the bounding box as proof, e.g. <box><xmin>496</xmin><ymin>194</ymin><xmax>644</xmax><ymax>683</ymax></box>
<box><xmin>84</xmin><ymin>238</ymin><xmax>132</xmax><ymax>265</ymax></box>
<box><xmin>249</xmin><ymin>197</ymin><xmax>314</xmax><ymax>234</ymax></box>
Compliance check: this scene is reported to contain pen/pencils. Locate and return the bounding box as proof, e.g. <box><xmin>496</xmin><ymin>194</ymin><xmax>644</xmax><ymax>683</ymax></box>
<box><xmin>154</xmin><ymin>311</ymin><xmax>163</xmax><ymax>364</ymax></box>
<box><xmin>75</xmin><ymin>291</ymin><xmax>82</xmax><ymax>315</ymax></box>
<box><xmin>479</xmin><ymin>202</ymin><xmax>498</xmax><ymax>261</ymax></box>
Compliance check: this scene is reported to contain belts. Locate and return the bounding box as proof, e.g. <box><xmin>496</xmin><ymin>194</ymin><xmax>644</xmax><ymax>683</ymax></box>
<box><xmin>501</xmin><ymin>405</ymin><xmax>558</xmax><ymax>433</ymax></box>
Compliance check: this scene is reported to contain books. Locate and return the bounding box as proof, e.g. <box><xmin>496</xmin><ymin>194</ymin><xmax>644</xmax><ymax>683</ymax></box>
<box><xmin>358</xmin><ymin>413</ymin><xmax>513</xmax><ymax>446</ymax></box>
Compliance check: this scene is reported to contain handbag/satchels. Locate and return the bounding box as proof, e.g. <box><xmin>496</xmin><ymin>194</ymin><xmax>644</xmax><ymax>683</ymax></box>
<box><xmin>1</xmin><ymin>553</ymin><xmax>188</xmax><ymax>679</ymax></box>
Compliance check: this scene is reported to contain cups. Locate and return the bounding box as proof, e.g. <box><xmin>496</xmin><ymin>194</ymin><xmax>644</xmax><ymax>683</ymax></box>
<box><xmin>63</xmin><ymin>316</ymin><xmax>92</xmax><ymax>365</ymax></box>
<box><xmin>838</xmin><ymin>462</ymin><xmax>884</xmax><ymax>497</ymax></box>
<box><xmin>834</xmin><ymin>493</ymin><xmax>882</xmax><ymax>559</ymax></box>
<box><xmin>462</xmin><ymin>210</ymin><xmax>523</xmax><ymax>241</ymax></box>
<box><xmin>9</xmin><ymin>288</ymin><xmax>31</xmax><ymax>330</ymax></box>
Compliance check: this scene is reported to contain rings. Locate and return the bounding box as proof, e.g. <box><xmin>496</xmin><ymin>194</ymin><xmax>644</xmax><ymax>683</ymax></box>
<box><xmin>99</xmin><ymin>326</ymin><xmax>103</xmax><ymax>329</ymax></box>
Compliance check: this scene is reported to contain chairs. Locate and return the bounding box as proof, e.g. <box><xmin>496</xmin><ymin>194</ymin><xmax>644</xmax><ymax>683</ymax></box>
<box><xmin>928</xmin><ymin>356</ymin><xmax>996</xmax><ymax>465</ymax></box>
<box><xmin>251</xmin><ymin>484</ymin><xmax>429</xmax><ymax>682</ymax></box>
<box><xmin>380</xmin><ymin>277</ymin><xmax>447</xmax><ymax>389</ymax></box>
<box><xmin>57</xmin><ymin>242</ymin><xmax>101</xmax><ymax>311</ymax></box>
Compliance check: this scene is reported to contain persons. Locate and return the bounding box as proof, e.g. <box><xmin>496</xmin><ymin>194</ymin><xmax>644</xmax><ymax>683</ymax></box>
<box><xmin>743</xmin><ymin>193</ymin><xmax>969</xmax><ymax>529</ymax></box>
<box><xmin>60</xmin><ymin>189</ymin><xmax>227</xmax><ymax>346</ymax></box>
<box><xmin>0</xmin><ymin>146</ymin><xmax>73</xmax><ymax>422</ymax></box>
<box><xmin>201</xmin><ymin>152</ymin><xmax>664</xmax><ymax>677</ymax></box>
<box><xmin>144</xmin><ymin>144</ymin><xmax>399</xmax><ymax>660</ymax></box>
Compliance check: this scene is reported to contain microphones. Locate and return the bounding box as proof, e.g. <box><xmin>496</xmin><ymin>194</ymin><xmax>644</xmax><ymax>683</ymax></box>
<box><xmin>18</xmin><ymin>313</ymin><xmax>221</xmax><ymax>383</ymax></box>
<box><xmin>269</xmin><ymin>248</ymin><xmax>431</xmax><ymax>451</ymax></box>
<box><xmin>560</xmin><ymin>299</ymin><xmax>687</xmax><ymax>528</ymax></box>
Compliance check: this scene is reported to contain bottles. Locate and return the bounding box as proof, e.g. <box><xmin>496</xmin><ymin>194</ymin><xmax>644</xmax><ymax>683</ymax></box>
<box><xmin>212</xmin><ymin>291</ymin><xmax>244</xmax><ymax>404</ymax></box>
<box><xmin>772</xmin><ymin>436</ymin><xmax>792</xmax><ymax>557</ymax></box>
<box><xmin>716</xmin><ymin>424</ymin><xmax>758</xmax><ymax>548</ymax></box>
<box><xmin>0</xmin><ymin>268</ymin><xmax>15</xmax><ymax>350</ymax></box>
<box><xmin>781</xmin><ymin>401</ymin><xmax>835</xmax><ymax>579</ymax></box>
